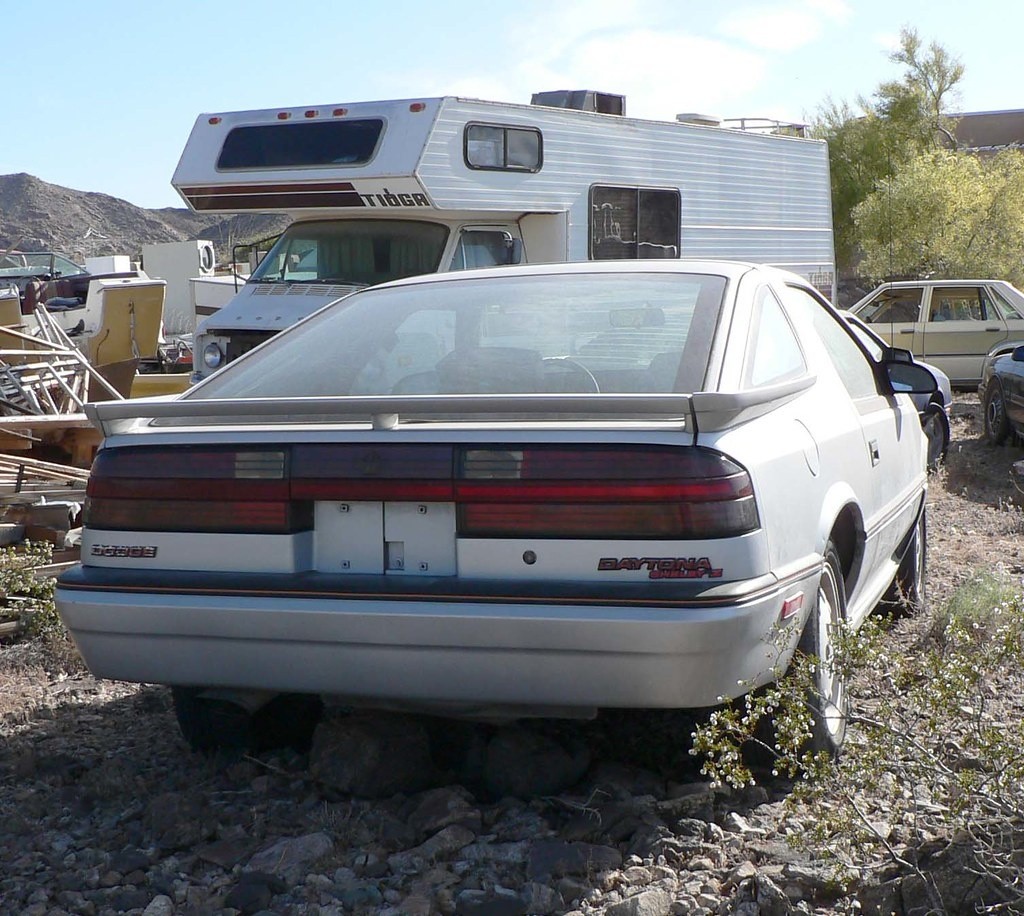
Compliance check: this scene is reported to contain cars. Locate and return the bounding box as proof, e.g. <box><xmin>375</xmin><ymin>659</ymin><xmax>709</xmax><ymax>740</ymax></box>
<box><xmin>977</xmin><ymin>342</ymin><xmax>1024</xmax><ymax>447</ymax></box>
<box><xmin>53</xmin><ymin>264</ymin><xmax>930</xmax><ymax>757</ymax></box>
<box><xmin>848</xmin><ymin>278</ymin><xmax>1024</xmax><ymax>389</ymax></box>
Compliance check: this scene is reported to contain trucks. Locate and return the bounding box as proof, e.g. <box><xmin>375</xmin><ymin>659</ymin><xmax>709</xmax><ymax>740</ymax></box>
<box><xmin>177</xmin><ymin>87</ymin><xmax>839</xmax><ymax>389</ymax></box>
